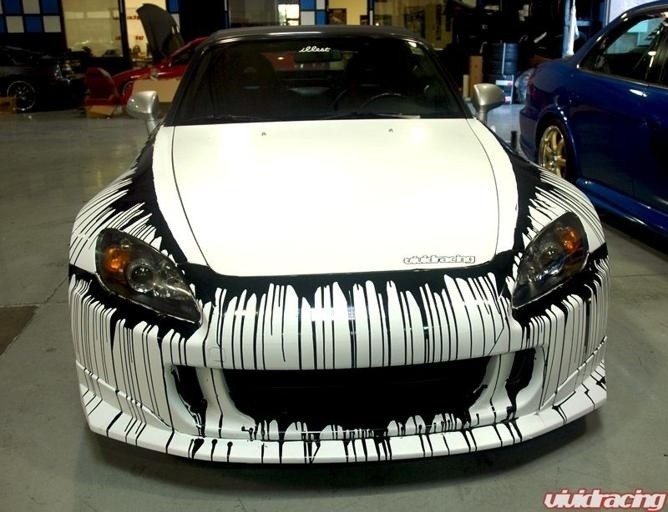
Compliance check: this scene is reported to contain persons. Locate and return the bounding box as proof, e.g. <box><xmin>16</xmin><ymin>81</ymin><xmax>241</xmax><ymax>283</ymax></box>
<box><xmin>131</xmin><ymin>45</ymin><xmax>145</xmax><ymax>59</ymax></box>
<box><xmin>507</xmin><ymin>1</ymin><xmax>544</xmax><ymax>75</ymax></box>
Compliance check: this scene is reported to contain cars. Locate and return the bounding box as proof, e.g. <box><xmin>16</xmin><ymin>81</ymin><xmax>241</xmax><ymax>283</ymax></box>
<box><xmin>67</xmin><ymin>22</ymin><xmax>610</xmax><ymax>470</ymax></box>
<box><xmin>1</xmin><ymin>43</ymin><xmax>92</xmax><ymax>111</ymax></box>
<box><xmin>518</xmin><ymin>0</ymin><xmax>668</xmax><ymax>234</ymax></box>
<box><xmin>84</xmin><ymin>37</ymin><xmax>207</xmax><ymax>117</ymax></box>
